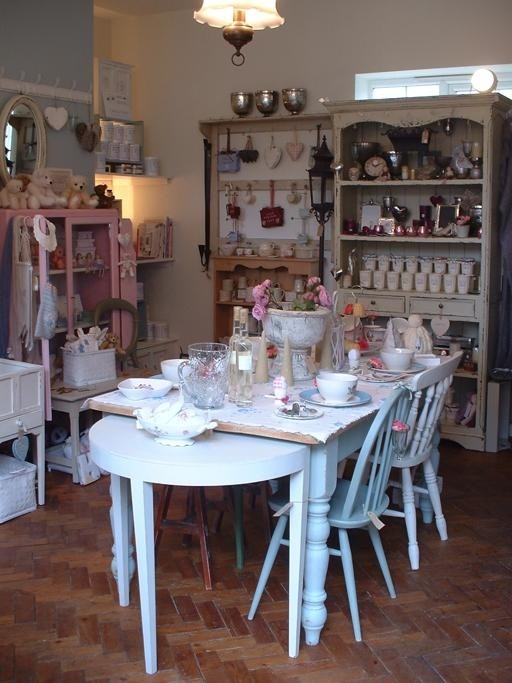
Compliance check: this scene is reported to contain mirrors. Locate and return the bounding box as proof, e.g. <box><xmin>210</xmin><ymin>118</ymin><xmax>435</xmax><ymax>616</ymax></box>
<box><xmin>0</xmin><ymin>89</ymin><xmax>49</xmax><ymax>187</ymax></box>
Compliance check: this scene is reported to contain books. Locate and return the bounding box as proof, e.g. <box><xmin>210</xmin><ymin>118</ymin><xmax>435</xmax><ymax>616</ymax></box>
<box><xmin>137</xmin><ymin>216</ymin><xmax>173</xmax><ymax>259</ymax></box>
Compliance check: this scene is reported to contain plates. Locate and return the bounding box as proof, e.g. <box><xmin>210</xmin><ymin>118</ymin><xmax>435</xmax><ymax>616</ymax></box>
<box><xmin>302</xmin><ymin>388</ymin><xmax>373</xmax><ymax>407</ymax></box>
<box><xmin>371</xmin><ymin>362</ymin><xmax>426</xmax><ymax>372</ymax></box>
<box><xmin>277</xmin><ymin>403</ymin><xmax>324</xmax><ymax>419</ymax></box>
<box><xmin>118</xmin><ymin>374</ymin><xmax>172</xmax><ymax>399</ymax></box>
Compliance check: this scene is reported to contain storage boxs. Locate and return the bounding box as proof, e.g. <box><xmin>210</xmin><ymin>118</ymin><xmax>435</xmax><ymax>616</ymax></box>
<box><xmin>0</xmin><ymin>451</ymin><xmax>41</xmax><ymax>525</ymax></box>
<box><xmin>62</xmin><ymin>347</ymin><xmax>121</xmax><ymax>387</ymax></box>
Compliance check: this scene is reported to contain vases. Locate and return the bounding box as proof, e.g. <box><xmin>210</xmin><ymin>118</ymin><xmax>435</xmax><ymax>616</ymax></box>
<box><xmin>263</xmin><ymin>304</ymin><xmax>332</xmax><ymax>379</ymax></box>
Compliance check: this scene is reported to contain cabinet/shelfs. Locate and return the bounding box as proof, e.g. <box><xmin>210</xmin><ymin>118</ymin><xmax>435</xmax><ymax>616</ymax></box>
<box><xmin>196</xmin><ymin>107</ymin><xmax>334</xmax><ymax>349</ymax></box>
<box><xmin>97</xmin><ymin>168</ymin><xmax>181</xmax><ymax>381</ymax></box>
<box><xmin>0</xmin><ymin>204</ymin><xmax>124</xmax><ymax>379</ymax></box>
<box><xmin>314</xmin><ymin>90</ymin><xmax>510</xmax><ymax>453</ymax></box>
<box><xmin>94</xmin><ymin>111</ymin><xmax>146</xmax><ymax>175</ymax></box>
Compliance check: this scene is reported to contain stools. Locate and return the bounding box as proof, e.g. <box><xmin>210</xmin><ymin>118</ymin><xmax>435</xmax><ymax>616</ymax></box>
<box><xmin>212</xmin><ymin>477</ymin><xmax>274</xmax><ymax>543</ymax></box>
<box><xmin>150</xmin><ymin>467</ymin><xmax>251</xmax><ymax>593</ymax></box>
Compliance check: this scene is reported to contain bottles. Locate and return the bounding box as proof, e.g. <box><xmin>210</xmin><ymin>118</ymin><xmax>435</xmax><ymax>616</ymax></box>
<box><xmin>229</xmin><ymin>306</ymin><xmax>253</xmax><ymax>409</ymax></box>
<box><xmin>409</xmin><ymin>168</ymin><xmax>415</xmax><ymax>179</ymax></box>
<box><xmin>401</xmin><ymin>166</ymin><xmax>408</xmax><ymax>179</ymax></box>
<box><xmin>348</xmin><ymin>348</ymin><xmax>360</xmax><ymax>368</ymax></box>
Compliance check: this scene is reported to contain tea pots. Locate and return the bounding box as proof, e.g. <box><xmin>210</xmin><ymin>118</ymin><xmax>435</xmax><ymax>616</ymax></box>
<box><xmin>254</xmin><ymin>240</ymin><xmax>274</xmax><ymax>256</ymax></box>
<box><xmin>219</xmin><ymin>243</ymin><xmax>236</xmax><ymax>255</ymax></box>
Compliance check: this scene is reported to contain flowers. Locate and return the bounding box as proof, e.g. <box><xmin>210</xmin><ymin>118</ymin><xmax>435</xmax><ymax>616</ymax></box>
<box><xmin>247</xmin><ymin>275</ymin><xmax>332</xmax><ymax>321</ymax></box>
<box><xmin>389</xmin><ymin>418</ymin><xmax>409</xmax><ymax>458</ymax></box>
<box><xmin>367</xmin><ymin>356</ymin><xmax>384</xmax><ymax>371</ymax></box>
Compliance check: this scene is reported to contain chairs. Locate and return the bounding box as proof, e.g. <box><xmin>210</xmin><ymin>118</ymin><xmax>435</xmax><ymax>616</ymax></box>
<box><xmin>337</xmin><ymin>344</ymin><xmax>465</xmax><ymax>573</ymax></box>
<box><xmin>238</xmin><ymin>385</ymin><xmax>410</xmax><ymax>645</ymax></box>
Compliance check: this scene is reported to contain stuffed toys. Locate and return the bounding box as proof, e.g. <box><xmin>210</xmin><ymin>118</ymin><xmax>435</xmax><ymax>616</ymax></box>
<box><xmin>0</xmin><ymin>180</ymin><xmax>28</xmax><ymax>210</ymax></box>
<box><xmin>100</xmin><ymin>332</ymin><xmax>126</xmax><ymax>355</ymax></box>
<box><xmin>25</xmin><ymin>168</ymin><xmax>67</xmax><ymax>208</ymax></box>
<box><xmin>73</xmin><ymin>252</ymin><xmax>105</xmax><ymax>280</ymax></box>
<box><xmin>52</xmin><ymin>246</ymin><xmax>66</xmax><ymax>268</ymax></box>
<box><xmin>119</xmin><ymin>254</ymin><xmax>137</xmax><ymax>278</ymax></box>
<box><xmin>62</xmin><ymin>175</ymin><xmax>99</xmax><ymax>209</ymax></box>
<box><xmin>91</xmin><ymin>185</ymin><xmax>114</xmax><ymax>209</ymax></box>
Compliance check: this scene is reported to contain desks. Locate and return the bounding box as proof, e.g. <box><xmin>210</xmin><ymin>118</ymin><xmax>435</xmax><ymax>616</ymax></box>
<box><xmin>0</xmin><ymin>351</ymin><xmax>57</xmax><ymax>525</ymax></box>
<box><xmin>29</xmin><ymin>366</ymin><xmax>156</xmax><ymax>485</ymax></box>
<box><xmin>80</xmin><ymin>331</ymin><xmax>445</xmax><ymax>677</ymax></box>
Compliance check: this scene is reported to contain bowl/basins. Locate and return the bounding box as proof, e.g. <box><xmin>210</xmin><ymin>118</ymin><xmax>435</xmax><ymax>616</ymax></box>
<box><xmin>452</xmin><ymin>167</ymin><xmax>469</xmax><ymax>178</ymax></box>
<box><xmin>162</xmin><ymin>358</ymin><xmax>188</xmax><ymax>386</ymax></box>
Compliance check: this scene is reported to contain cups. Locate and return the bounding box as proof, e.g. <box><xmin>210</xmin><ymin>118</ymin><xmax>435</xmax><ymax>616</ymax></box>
<box><xmin>315</xmin><ymin>373</ymin><xmax>358</xmax><ymax>401</ymax></box>
<box><xmin>359</xmin><ymin>254</ymin><xmax>474</xmax><ymax>292</ymax></box>
<box><xmin>178</xmin><ymin>342</ymin><xmax>229</xmax><ymax>410</ymax></box>
<box><xmin>236</xmin><ymin>248</ymin><xmax>252</xmax><ymax>255</ymax></box>
<box><xmin>378</xmin><ymin>347</ymin><xmax>414</xmax><ymax>371</ymax></box>
<box><xmin>342</xmin><ymin>316</ymin><xmax>363</xmax><ymax>340</ymax></box>
<box><xmin>144</xmin><ymin>155</ymin><xmax>159</xmax><ymax>176</ymax></box>
<box><xmin>101</xmin><ymin>122</ymin><xmax>141</xmax><ymax>160</ymax></box>
<box><xmin>456</xmin><ymin>224</ymin><xmax>470</xmax><ymax>237</ymax></box>
<box><xmin>219</xmin><ymin>277</ymin><xmax>305</xmax><ymax>302</ymax></box>
<box><xmin>95</xmin><ymin>151</ymin><xmax>106</xmax><ymax>172</ymax></box>
<box><xmin>363</xmin><ymin>325</ymin><xmax>387</xmax><ymax>343</ymax></box>
<box><xmin>117</xmin><ymin>164</ymin><xmax>143</xmax><ymax>173</ymax></box>
<box><xmin>281</xmin><ymin>246</ymin><xmax>294</xmax><ymax>257</ymax></box>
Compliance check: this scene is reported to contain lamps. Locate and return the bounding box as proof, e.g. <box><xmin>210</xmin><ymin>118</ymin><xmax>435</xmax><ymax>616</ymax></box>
<box><xmin>303</xmin><ymin>134</ymin><xmax>335</xmax><ymax>226</ymax></box>
<box><xmin>187</xmin><ymin>0</ymin><xmax>284</xmax><ymax>65</ymax></box>
<box><xmin>471</xmin><ymin>65</ymin><xmax>498</xmax><ymax>90</ymax></box>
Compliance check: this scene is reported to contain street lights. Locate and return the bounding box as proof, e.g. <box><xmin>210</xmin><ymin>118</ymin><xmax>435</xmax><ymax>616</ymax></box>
<box><xmin>302</xmin><ymin>131</ymin><xmax>337</xmax><ymax>284</ymax></box>
